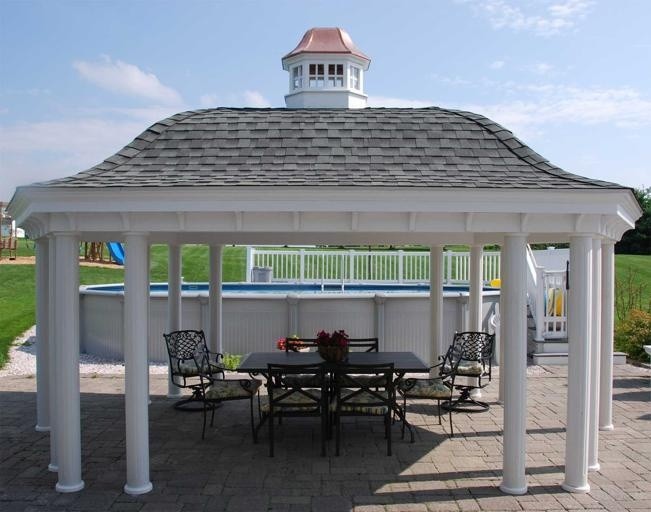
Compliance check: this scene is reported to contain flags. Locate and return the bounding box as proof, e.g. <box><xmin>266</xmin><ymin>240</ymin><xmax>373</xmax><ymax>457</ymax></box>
<box><xmin>288</xmin><ymin>347</ymin><xmax>311</xmax><ymax>352</ymax></box>
<box><xmin>319</xmin><ymin>346</ymin><xmax>349</xmax><ymax>363</ymax></box>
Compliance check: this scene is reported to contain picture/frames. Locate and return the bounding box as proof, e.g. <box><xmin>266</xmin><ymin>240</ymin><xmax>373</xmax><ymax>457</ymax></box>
<box><xmin>235</xmin><ymin>351</ymin><xmax>430</xmax><ymax>442</ymax></box>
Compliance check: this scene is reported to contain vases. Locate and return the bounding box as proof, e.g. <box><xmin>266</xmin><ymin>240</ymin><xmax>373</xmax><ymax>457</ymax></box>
<box><xmin>315</xmin><ymin>329</ymin><xmax>350</xmax><ymax>346</ymax></box>
<box><xmin>275</xmin><ymin>334</ymin><xmax>308</xmax><ymax>351</ymax></box>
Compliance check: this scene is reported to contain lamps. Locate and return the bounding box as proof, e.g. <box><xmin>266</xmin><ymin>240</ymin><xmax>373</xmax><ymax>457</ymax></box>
<box><xmin>276</xmin><ymin>337</ymin><xmax>323</xmax><ymax>428</ymax></box>
<box><xmin>191</xmin><ymin>343</ymin><xmax>262</xmax><ymax>440</ymax></box>
<box><xmin>260</xmin><ymin>361</ymin><xmax>330</xmax><ymax>457</ymax></box>
<box><xmin>163</xmin><ymin>330</ymin><xmax>226</xmax><ymax>412</ymax></box>
<box><xmin>438</xmin><ymin>332</ymin><xmax>496</xmax><ymax>414</ymax></box>
<box><xmin>333</xmin><ymin>337</ymin><xmax>390</xmax><ymax>424</ymax></box>
<box><xmin>397</xmin><ymin>344</ymin><xmax>468</xmax><ymax>439</ymax></box>
<box><xmin>332</xmin><ymin>362</ymin><xmax>395</xmax><ymax>456</ymax></box>
